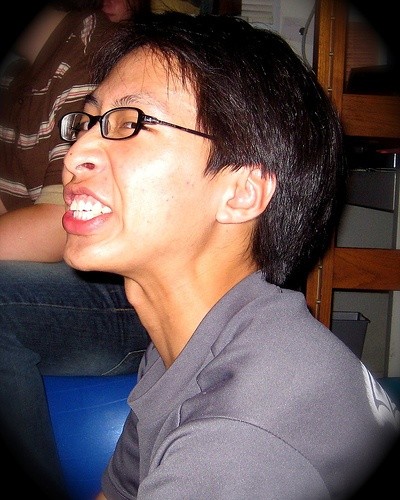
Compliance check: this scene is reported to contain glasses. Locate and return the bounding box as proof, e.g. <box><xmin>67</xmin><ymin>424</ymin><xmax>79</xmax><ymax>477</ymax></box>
<box><xmin>59</xmin><ymin>107</ymin><xmax>219</xmax><ymax>142</ymax></box>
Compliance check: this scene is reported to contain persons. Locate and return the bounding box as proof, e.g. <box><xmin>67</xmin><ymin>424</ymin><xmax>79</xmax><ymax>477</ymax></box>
<box><xmin>0</xmin><ymin>0</ymin><xmax>153</xmax><ymax>500</ymax></box>
<box><xmin>57</xmin><ymin>6</ymin><xmax>400</xmax><ymax>500</ymax></box>
